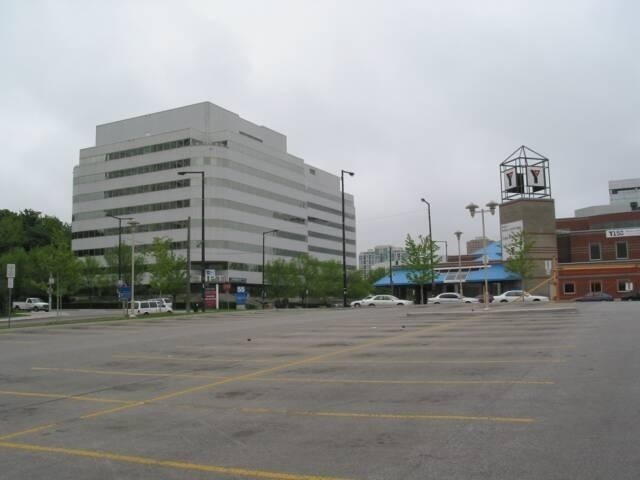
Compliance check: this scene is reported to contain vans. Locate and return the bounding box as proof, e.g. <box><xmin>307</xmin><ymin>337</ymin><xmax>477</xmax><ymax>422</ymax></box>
<box><xmin>126</xmin><ymin>297</ymin><xmax>173</xmax><ymax>317</ymax></box>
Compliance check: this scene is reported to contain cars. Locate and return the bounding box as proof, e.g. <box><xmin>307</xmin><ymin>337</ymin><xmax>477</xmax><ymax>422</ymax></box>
<box><xmin>427</xmin><ymin>292</ymin><xmax>479</xmax><ymax>304</ymax></box>
<box><xmin>584</xmin><ymin>292</ymin><xmax>613</xmax><ymax>301</ymax></box>
<box><xmin>493</xmin><ymin>289</ymin><xmax>549</xmax><ymax>303</ymax></box>
<box><xmin>621</xmin><ymin>292</ymin><xmax>640</xmax><ymax>301</ymax></box>
<box><xmin>351</xmin><ymin>294</ymin><xmax>413</xmax><ymax>308</ymax></box>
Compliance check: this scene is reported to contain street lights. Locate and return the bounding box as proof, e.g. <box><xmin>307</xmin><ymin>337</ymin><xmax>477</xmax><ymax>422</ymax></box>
<box><xmin>455</xmin><ymin>232</ymin><xmax>462</xmax><ymax>303</ymax></box>
<box><xmin>178</xmin><ymin>172</ymin><xmax>205</xmax><ymax>312</ymax></box>
<box><xmin>422</xmin><ymin>199</ymin><xmax>435</xmax><ymax>296</ymax></box>
<box><xmin>342</xmin><ymin>170</ymin><xmax>354</xmax><ymax>306</ymax></box>
<box><xmin>127</xmin><ymin>221</ymin><xmax>139</xmax><ymax>318</ymax></box>
<box><xmin>466</xmin><ymin>201</ymin><xmax>498</xmax><ymax>308</ymax></box>
<box><xmin>262</xmin><ymin>229</ymin><xmax>277</xmax><ymax>309</ymax></box>
<box><xmin>106</xmin><ymin>214</ymin><xmax>132</xmax><ymax>301</ymax></box>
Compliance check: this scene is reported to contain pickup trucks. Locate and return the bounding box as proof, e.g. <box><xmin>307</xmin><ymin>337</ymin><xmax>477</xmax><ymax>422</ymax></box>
<box><xmin>12</xmin><ymin>298</ymin><xmax>49</xmax><ymax>312</ymax></box>
